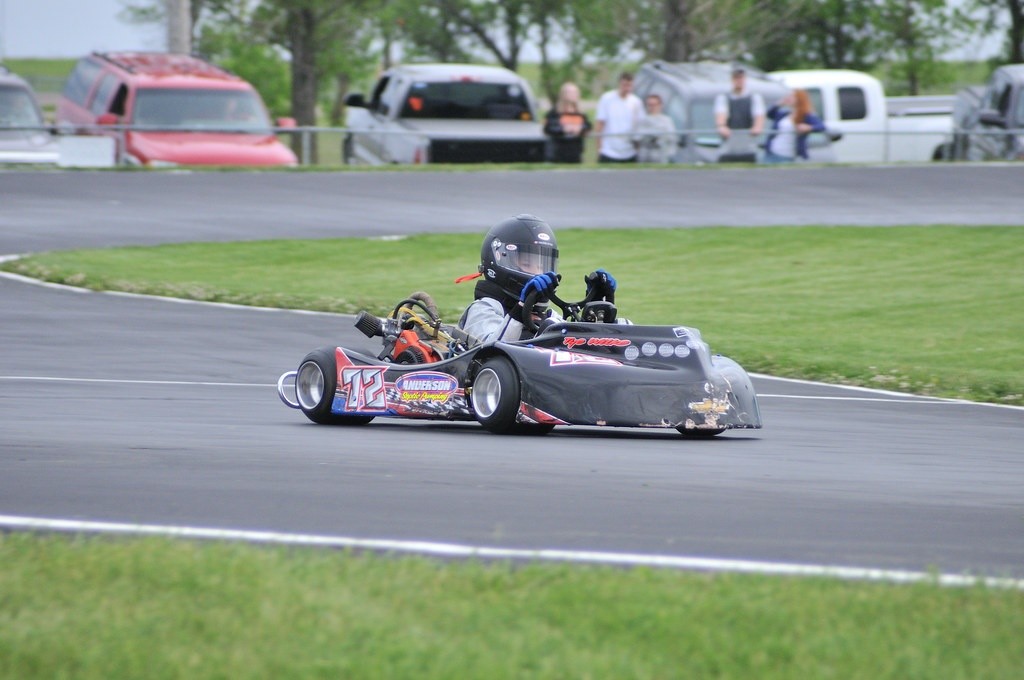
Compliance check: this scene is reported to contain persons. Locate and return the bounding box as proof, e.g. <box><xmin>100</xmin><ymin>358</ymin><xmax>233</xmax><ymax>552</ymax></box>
<box><xmin>594</xmin><ymin>73</ymin><xmax>646</xmax><ymax>161</ymax></box>
<box><xmin>714</xmin><ymin>69</ymin><xmax>765</xmax><ymax>141</ymax></box>
<box><xmin>454</xmin><ymin>214</ymin><xmax>616</xmax><ymax>342</ymax></box>
<box><xmin>545</xmin><ymin>83</ymin><xmax>591</xmax><ymax>161</ymax></box>
<box><xmin>639</xmin><ymin>94</ymin><xmax>678</xmax><ymax>163</ymax></box>
<box><xmin>765</xmin><ymin>89</ymin><xmax>824</xmax><ymax>162</ymax></box>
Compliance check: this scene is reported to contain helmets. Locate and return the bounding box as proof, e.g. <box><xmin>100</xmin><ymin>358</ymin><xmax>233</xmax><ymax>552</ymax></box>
<box><xmin>478</xmin><ymin>213</ymin><xmax>558</xmax><ymax>302</ymax></box>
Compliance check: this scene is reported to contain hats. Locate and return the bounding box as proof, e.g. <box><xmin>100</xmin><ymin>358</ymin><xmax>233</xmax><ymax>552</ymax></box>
<box><xmin>732</xmin><ymin>61</ymin><xmax>745</xmax><ymax>76</ymax></box>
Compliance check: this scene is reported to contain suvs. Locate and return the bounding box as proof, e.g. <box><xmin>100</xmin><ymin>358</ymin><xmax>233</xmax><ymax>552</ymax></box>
<box><xmin>630</xmin><ymin>60</ymin><xmax>843</xmax><ymax>162</ymax></box>
<box><xmin>55</xmin><ymin>52</ymin><xmax>299</xmax><ymax>167</ymax></box>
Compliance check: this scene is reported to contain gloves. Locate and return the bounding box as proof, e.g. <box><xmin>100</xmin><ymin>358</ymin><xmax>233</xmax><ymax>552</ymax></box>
<box><xmin>584</xmin><ymin>269</ymin><xmax>616</xmax><ymax>296</ymax></box>
<box><xmin>520</xmin><ymin>271</ymin><xmax>562</xmax><ymax>305</ymax></box>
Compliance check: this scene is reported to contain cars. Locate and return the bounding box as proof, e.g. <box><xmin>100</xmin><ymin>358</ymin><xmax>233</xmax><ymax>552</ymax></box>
<box><xmin>0</xmin><ymin>64</ymin><xmax>77</xmax><ymax>141</ymax></box>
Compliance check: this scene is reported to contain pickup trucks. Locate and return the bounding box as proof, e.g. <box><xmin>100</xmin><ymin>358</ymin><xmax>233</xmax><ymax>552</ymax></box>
<box><xmin>341</xmin><ymin>64</ymin><xmax>556</xmax><ymax>165</ymax></box>
<box><xmin>766</xmin><ymin>70</ymin><xmax>959</xmax><ymax>163</ymax></box>
<box><xmin>953</xmin><ymin>63</ymin><xmax>1024</xmax><ymax>161</ymax></box>
